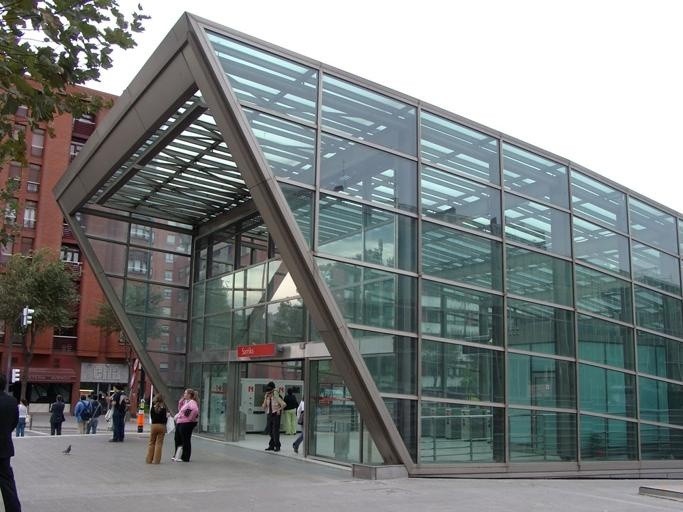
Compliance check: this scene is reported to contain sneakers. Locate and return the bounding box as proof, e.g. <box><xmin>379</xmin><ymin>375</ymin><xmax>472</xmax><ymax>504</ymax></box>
<box><xmin>293</xmin><ymin>442</ymin><xmax>299</xmax><ymax>452</ymax></box>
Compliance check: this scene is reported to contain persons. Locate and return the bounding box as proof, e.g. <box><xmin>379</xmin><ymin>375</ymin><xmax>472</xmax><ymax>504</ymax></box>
<box><xmin>171</xmin><ymin>389</ymin><xmax>200</xmax><ymax>462</ymax></box>
<box><xmin>283</xmin><ymin>388</ymin><xmax>298</xmax><ymax>435</ymax></box>
<box><xmin>261</xmin><ymin>382</ymin><xmax>287</xmax><ymax>451</ymax></box>
<box><xmin>15</xmin><ymin>399</ymin><xmax>28</xmax><ymax>437</ymax></box>
<box><xmin>49</xmin><ymin>395</ymin><xmax>65</xmax><ymax>435</ymax></box>
<box><xmin>292</xmin><ymin>395</ymin><xmax>305</xmax><ymax>455</ymax></box>
<box><xmin>0</xmin><ymin>374</ymin><xmax>22</xmax><ymax>512</ymax></box>
<box><xmin>74</xmin><ymin>383</ymin><xmax>130</xmax><ymax>443</ymax></box>
<box><xmin>145</xmin><ymin>392</ymin><xmax>170</xmax><ymax>465</ymax></box>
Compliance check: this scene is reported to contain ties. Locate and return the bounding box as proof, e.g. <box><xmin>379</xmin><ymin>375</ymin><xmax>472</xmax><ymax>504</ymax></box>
<box><xmin>269</xmin><ymin>397</ymin><xmax>272</xmax><ymax>414</ymax></box>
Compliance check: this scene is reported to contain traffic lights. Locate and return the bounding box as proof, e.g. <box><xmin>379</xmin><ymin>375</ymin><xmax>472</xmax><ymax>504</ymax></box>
<box><xmin>15</xmin><ymin>369</ymin><xmax>20</xmax><ymax>382</ymax></box>
<box><xmin>26</xmin><ymin>308</ymin><xmax>34</xmax><ymax>325</ymax></box>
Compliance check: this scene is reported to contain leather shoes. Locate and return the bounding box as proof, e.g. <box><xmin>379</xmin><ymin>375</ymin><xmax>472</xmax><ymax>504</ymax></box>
<box><xmin>265</xmin><ymin>446</ymin><xmax>281</xmax><ymax>452</ymax></box>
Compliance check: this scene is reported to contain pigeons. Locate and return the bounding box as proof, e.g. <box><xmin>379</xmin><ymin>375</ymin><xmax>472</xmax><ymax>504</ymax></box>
<box><xmin>61</xmin><ymin>444</ymin><xmax>72</xmax><ymax>455</ymax></box>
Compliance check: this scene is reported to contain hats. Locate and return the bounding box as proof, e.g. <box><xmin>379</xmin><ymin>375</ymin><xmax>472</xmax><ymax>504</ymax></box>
<box><xmin>266</xmin><ymin>386</ymin><xmax>273</xmax><ymax>393</ymax></box>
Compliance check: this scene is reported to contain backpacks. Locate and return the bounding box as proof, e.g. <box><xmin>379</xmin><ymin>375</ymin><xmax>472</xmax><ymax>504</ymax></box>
<box><xmin>79</xmin><ymin>399</ymin><xmax>93</xmax><ymax>421</ymax></box>
<box><xmin>117</xmin><ymin>390</ymin><xmax>131</xmax><ymax>412</ymax></box>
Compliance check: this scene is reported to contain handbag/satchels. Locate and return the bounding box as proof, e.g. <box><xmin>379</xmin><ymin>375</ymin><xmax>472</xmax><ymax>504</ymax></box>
<box><xmin>184</xmin><ymin>408</ymin><xmax>198</xmax><ymax>418</ymax></box>
